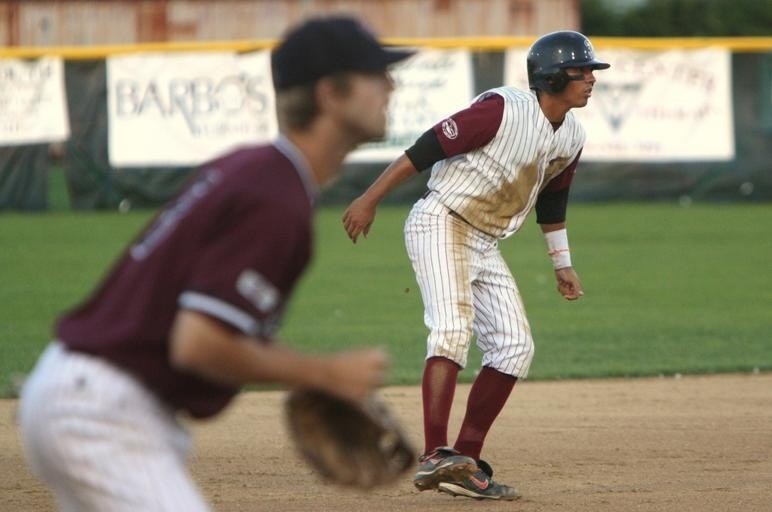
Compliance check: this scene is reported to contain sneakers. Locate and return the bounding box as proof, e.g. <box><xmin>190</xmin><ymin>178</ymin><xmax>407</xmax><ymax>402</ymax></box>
<box><xmin>413</xmin><ymin>446</ymin><xmax>478</xmax><ymax>491</ymax></box>
<box><xmin>438</xmin><ymin>459</ymin><xmax>517</xmax><ymax>502</ymax></box>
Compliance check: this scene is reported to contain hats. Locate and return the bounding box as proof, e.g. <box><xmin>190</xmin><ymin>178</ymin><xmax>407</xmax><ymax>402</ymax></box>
<box><xmin>268</xmin><ymin>17</ymin><xmax>416</xmax><ymax>90</ymax></box>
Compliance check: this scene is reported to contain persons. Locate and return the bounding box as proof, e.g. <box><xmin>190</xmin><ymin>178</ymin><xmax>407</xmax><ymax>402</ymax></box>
<box><xmin>18</xmin><ymin>16</ymin><xmax>419</xmax><ymax>511</ymax></box>
<box><xmin>340</xmin><ymin>31</ymin><xmax>609</xmax><ymax>501</ymax></box>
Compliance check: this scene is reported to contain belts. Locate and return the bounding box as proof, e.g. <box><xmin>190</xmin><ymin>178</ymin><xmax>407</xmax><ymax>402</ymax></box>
<box><xmin>421</xmin><ymin>190</ymin><xmax>468</xmax><ymax>225</ymax></box>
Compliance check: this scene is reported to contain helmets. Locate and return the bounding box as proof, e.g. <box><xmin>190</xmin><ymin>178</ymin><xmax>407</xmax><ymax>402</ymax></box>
<box><xmin>526</xmin><ymin>31</ymin><xmax>611</xmax><ymax>95</ymax></box>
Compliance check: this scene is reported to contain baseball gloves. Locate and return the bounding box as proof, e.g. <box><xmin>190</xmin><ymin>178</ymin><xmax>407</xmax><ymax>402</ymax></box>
<box><xmin>290</xmin><ymin>386</ymin><xmax>412</xmax><ymax>488</ymax></box>
